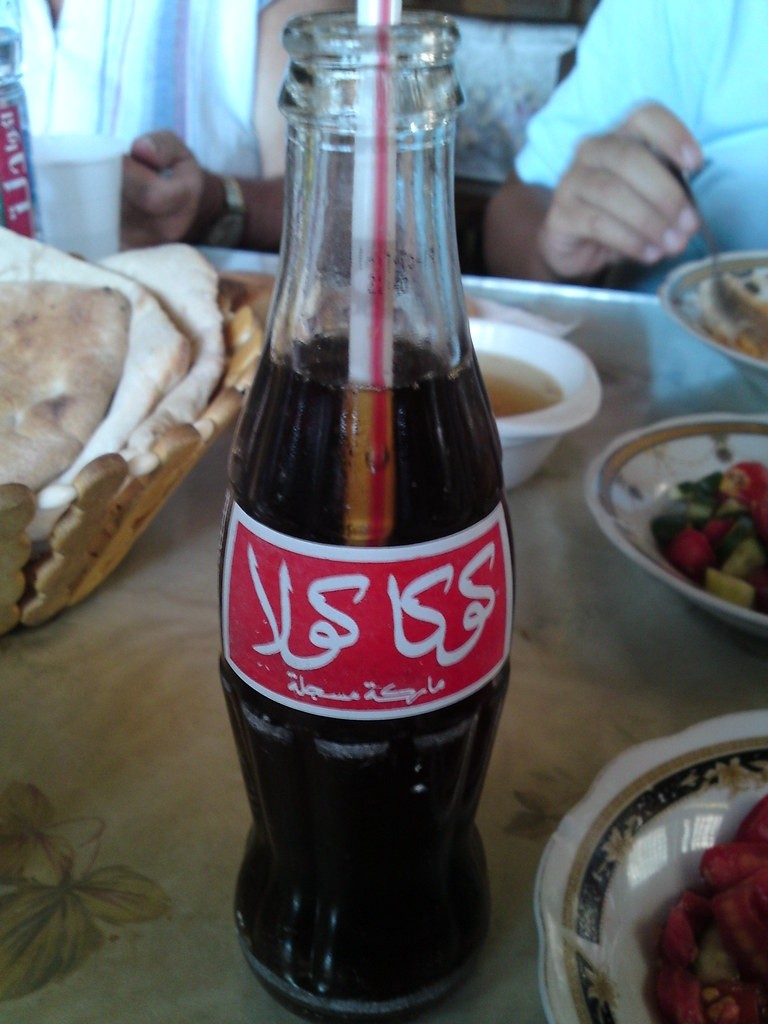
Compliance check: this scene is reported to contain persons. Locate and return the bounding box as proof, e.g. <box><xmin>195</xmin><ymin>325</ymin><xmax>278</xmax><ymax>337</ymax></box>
<box><xmin>481</xmin><ymin>0</ymin><xmax>768</xmax><ymax>299</ymax></box>
<box><xmin>0</xmin><ymin>0</ymin><xmax>356</xmax><ymax>265</ymax></box>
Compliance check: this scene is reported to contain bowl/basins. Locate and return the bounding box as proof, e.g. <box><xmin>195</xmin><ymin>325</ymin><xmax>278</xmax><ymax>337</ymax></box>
<box><xmin>582</xmin><ymin>413</ymin><xmax>768</xmax><ymax>637</ymax></box>
<box><xmin>662</xmin><ymin>249</ymin><xmax>768</xmax><ymax>377</ymax></box>
<box><xmin>463</xmin><ymin>314</ymin><xmax>602</xmax><ymax>500</ymax></box>
<box><xmin>533</xmin><ymin>709</ymin><xmax>768</xmax><ymax>1024</ymax></box>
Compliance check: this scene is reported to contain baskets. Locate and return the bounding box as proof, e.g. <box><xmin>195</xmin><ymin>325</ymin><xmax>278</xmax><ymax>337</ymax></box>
<box><xmin>0</xmin><ymin>296</ymin><xmax>262</xmax><ymax>634</ymax></box>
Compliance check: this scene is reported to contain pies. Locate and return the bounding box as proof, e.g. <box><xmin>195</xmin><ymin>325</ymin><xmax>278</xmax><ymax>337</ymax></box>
<box><xmin>0</xmin><ymin>224</ymin><xmax>227</xmax><ymax>539</ymax></box>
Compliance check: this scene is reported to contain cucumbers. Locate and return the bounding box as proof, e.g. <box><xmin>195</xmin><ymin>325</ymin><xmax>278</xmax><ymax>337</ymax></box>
<box><xmin>647</xmin><ymin>471</ymin><xmax>765</xmax><ymax>611</ymax></box>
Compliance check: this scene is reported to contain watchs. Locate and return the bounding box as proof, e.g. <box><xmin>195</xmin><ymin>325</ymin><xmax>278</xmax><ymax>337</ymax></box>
<box><xmin>208</xmin><ymin>174</ymin><xmax>247</xmax><ymax>248</ymax></box>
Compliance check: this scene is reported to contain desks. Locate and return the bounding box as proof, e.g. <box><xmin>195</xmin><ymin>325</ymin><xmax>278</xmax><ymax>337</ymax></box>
<box><xmin>0</xmin><ymin>249</ymin><xmax>768</xmax><ymax>1024</ymax></box>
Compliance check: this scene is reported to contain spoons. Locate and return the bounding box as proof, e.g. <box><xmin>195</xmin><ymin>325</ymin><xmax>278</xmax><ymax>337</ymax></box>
<box><xmin>666</xmin><ymin>155</ymin><xmax>764</xmax><ymax>340</ymax></box>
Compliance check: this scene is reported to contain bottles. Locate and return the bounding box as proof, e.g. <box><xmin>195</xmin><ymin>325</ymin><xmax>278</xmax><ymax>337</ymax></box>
<box><xmin>215</xmin><ymin>17</ymin><xmax>510</xmax><ymax>1024</ymax></box>
